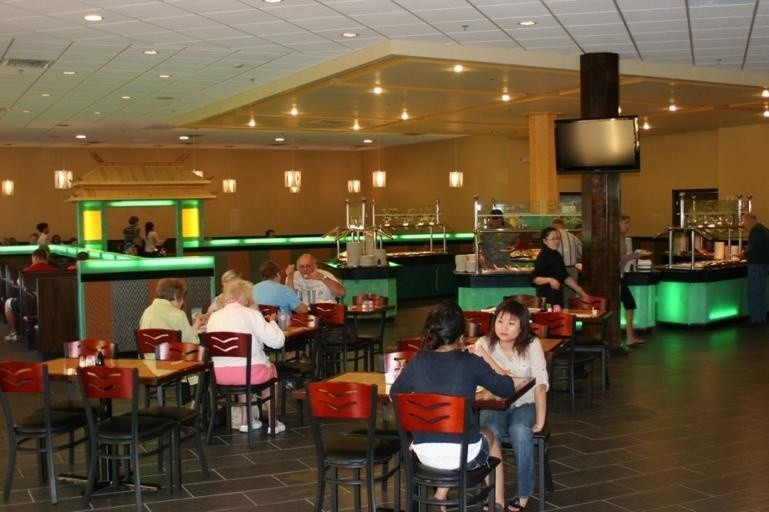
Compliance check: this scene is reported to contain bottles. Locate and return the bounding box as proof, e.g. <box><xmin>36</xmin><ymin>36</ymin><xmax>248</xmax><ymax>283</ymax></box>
<box><xmin>97</xmin><ymin>348</ymin><xmax>104</xmax><ymax>365</ymax></box>
<box><xmin>278</xmin><ymin>307</ymin><xmax>290</xmax><ymax>331</ymax></box>
<box><xmin>79</xmin><ymin>354</ymin><xmax>85</xmax><ymax>368</ymax></box>
<box><xmin>539</xmin><ymin>296</ymin><xmax>547</xmax><ymax>312</ymax></box>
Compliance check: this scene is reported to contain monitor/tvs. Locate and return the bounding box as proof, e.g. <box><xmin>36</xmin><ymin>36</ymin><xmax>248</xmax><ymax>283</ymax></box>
<box><xmin>553</xmin><ymin>115</ymin><xmax>641</xmax><ymax>174</ymax></box>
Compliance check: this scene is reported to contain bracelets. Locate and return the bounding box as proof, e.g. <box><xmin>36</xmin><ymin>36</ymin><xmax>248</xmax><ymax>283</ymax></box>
<box><xmin>322</xmin><ymin>275</ymin><xmax>328</xmax><ymax>281</ymax></box>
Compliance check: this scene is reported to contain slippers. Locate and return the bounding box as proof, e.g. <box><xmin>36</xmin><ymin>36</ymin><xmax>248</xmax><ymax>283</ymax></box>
<box><xmin>627</xmin><ymin>338</ymin><xmax>646</xmax><ymax>347</ymax></box>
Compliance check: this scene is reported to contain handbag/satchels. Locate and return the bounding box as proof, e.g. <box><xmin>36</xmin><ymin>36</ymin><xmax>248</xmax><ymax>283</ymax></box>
<box><xmin>318</xmin><ymin>327</ymin><xmax>356</xmax><ymax>345</ymax></box>
<box><xmin>231</xmin><ymin>394</ymin><xmax>259</xmax><ymax>429</ymax></box>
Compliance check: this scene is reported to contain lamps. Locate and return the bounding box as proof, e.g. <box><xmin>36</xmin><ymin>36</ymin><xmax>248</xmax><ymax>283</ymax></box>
<box><xmin>52</xmin><ymin>125</ymin><xmax>74</xmax><ymax>190</ymax></box>
<box><xmin>1</xmin><ymin>139</ymin><xmax>14</xmax><ymax>197</ymax></box>
<box><xmin>347</xmin><ymin>141</ymin><xmax>362</xmax><ymax>195</ymax></box>
<box><xmin>447</xmin><ymin>135</ymin><xmax>464</xmax><ymax>189</ymax></box>
<box><xmin>221</xmin><ymin>145</ymin><xmax>236</xmax><ymax>194</ymax></box>
<box><xmin>283</xmin><ymin>138</ymin><xmax>302</xmax><ymax>188</ymax></box>
<box><xmin>370</xmin><ymin>140</ymin><xmax>387</xmax><ymax>188</ymax></box>
<box><xmin>193</xmin><ymin>136</ymin><xmax>204</xmax><ymax>177</ymax></box>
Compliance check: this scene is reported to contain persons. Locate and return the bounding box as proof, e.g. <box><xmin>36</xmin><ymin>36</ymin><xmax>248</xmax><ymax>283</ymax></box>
<box><xmin>737</xmin><ymin>212</ymin><xmax>768</xmax><ymax>326</ymax></box>
<box><xmin>68</xmin><ymin>249</ymin><xmax>90</xmax><ymax>273</ymax></box>
<box><xmin>473</xmin><ymin>208</ymin><xmax>521</xmax><ymax>269</ymax></box>
<box><xmin>618</xmin><ymin>215</ymin><xmax>647</xmax><ymax>347</ymax></box>
<box><xmin>68</xmin><ymin>235</ymin><xmax>79</xmax><ymax>245</ymax></box>
<box><xmin>143</xmin><ymin>220</ymin><xmax>166</xmax><ymax>256</ymax></box>
<box><xmin>1</xmin><ymin>248</ymin><xmax>58</xmax><ymax>343</ymax></box>
<box><xmin>527</xmin><ymin>226</ymin><xmax>589</xmax><ymax>311</ymax></box>
<box><xmin>50</xmin><ymin>234</ymin><xmax>63</xmax><ymax>243</ymax></box>
<box><xmin>199</xmin><ymin>269</ymin><xmax>243</xmax><ymax>317</ymax></box>
<box><xmin>135</xmin><ymin>275</ymin><xmax>212</xmax><ymax>427</ymax></box>
<box><xmin>204</xmin><ymin>279</ymin><xmax>286</xmax><ymax>435</ymax></box>
<box><xmin>387</xmin><ymin>302</ymin><xmax>516</xmax><ymax>512</ymax></box>
<box><xmin>121</xmin><ymin>214</ymin><xmax>143</xmax><ymax>254</ymax></box>
<box><xmin>33</xmin><ymin>220</ymin><xmax>52</xmax><ymax>246</ymax></box>
<box><xmin>282</xmin><ymin>252</ymin><xmax>346</xmax><ymax>367</ymax></box>
<box><xmin>472</xmin><ymin>300</ymin><xmax>551</xmax><ymax>512</ymax></box>
<box><xmin>263</xmin><ymin>228</ymin><xmax>276</xmax><ymax>242</ymax></box>
<box><xmin>549</xmin><ymin>217</ymin><xmax>584</xmax><ymax>308</ymax></box>
<box><xmin>250</xmin><ymin>260</ymin><xmax>309</xmax><ymax>391</ymax></box>
<box><xmin>37</xmin><ymin>243</ymin><xmax>62</xmax><ymax>265</ymax></box>
<box><xmin>28</xmin><ymin>232</ymin><xmax>39</xmax><ymax>246</ymax></box>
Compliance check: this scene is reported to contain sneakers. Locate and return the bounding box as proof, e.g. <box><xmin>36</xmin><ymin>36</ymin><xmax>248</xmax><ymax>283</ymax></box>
<box><xmin>239</xmin><ymin>420</ymin><xmax>262</xmax><ymax>432</ymax></box>
<box><xmin>267</xmin><ymin>420</ymin><xmax>286</xmax><ymax>435</ymax></box>
<box><xmin>5</xmin><ymin>334</ymin><xmax>18</xmax><ymax>343</ymax></box>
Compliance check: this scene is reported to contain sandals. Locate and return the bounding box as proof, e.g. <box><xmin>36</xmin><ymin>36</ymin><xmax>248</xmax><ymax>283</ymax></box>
<box><xmin>481</xmin><ymin>500</ymin><xmax>505</xmax><ymax>512</ymax></box>
<box><xmin>504</xmin><ymin>497</ymin><xmax>529</xmax><ymax>512</ymax></box>
<box><xmin>427</xmin><ymin>495</ymin><xmax>449</xmax><ymax>512</ymax></box>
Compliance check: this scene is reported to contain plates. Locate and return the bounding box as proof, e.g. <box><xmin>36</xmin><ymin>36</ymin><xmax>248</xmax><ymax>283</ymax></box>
<box><xmin>373</xmin><ymin>249</ymin><xmax>388</xmax><ymax>267</ymax></box>
<box><xmin>360</xmin><ymin>254</ymin><xmax>376</xmax><ymax>267</ymax></box>
<box><xmin>455</xmin><ymin>254</ymin><xmax>477</xmax><ymax>273</ymax></box>
<box><xmin>713</xmin><ymin>241</ymin><xmax>739</xmax><ymax>260</ymax></box>
<box><xmin>346</xmin><ymin>241</ymin><xmax>362</xmax><ymax>268</ymax></box>
